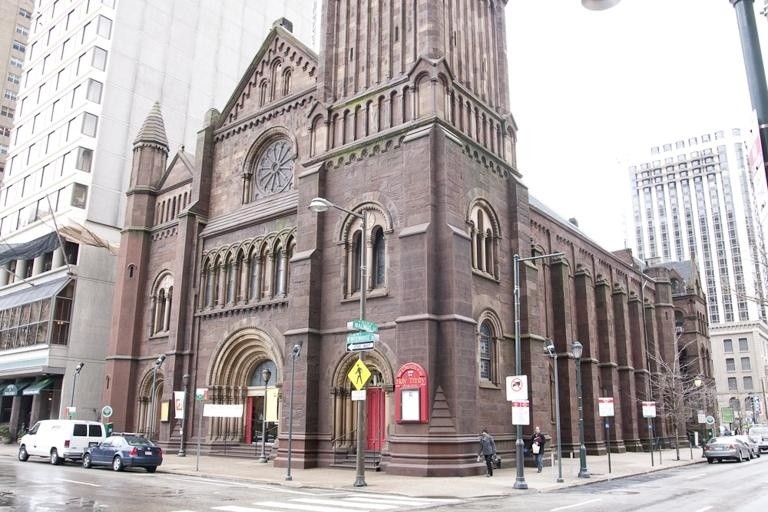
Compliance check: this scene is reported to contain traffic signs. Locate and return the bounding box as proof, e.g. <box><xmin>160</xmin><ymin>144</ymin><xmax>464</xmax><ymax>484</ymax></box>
<box><xmin>353</xmin><ymin>320</ymin><xmax>380</xmax><ymax>333</ymax></box>
<box><xmin>345</xmin><ymin>341</ymin><xmax>374</xmax><ymax>352</ymax></box>
<box><xmin>346</xmin><ymin>333</ymin><xmax>374</xmax><ymax>343</ymax></box>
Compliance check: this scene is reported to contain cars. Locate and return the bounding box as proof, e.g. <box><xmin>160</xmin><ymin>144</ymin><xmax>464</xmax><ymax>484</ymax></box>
<box><xmin>748</xmin><ymin>427</ymin><xmax>768</xmax><ymax>452</ymax></box>
<box><xmin>733</xmin><ymin>433</ymin><xmax>761</xmax><ymax>459</ymax></box>
<box><xmin>81</xmin><ymin>430</ymin><xmax>162</xmax><ymax>473</ymax></box>
<box><xmin>703</xmin><ymin>435</ymin><xmax>751</xmax><ymax>464</ymax></box>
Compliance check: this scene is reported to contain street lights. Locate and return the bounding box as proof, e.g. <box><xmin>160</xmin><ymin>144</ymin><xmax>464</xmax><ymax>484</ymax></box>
<box><xmin>737</xmin><ymin>410</ymin><xmax>743</xmax><ymax>428</ymax></box>
<box><xmin>149</xmin><ymin>353</ymin><xmax>166</xmax><ymax>440</ymax></box>
<box><xmin>70</xmin><ymin>361</ymin><xmax>84</xmax><ymax>420</ymax></box>
<box><xmin>571</xmin><ymin>341</ymin><xmax>591</xmax><ymax>479</ymax></box>
<box><xmin>284</xmin><ymin>339</ymin><xmax>305</xmax><ymax>481</ymax></box>
<box><xmin>256</xmin><ymin>367</ymin><xmax>272</xmax><ymax>464</ymax></box>
<box><xmin>176</xmin><ymin>371</ymin><xmax>192</xmax><ymax>457</ymax></box>
<box><xmin>694</xmin><ymin>373</ymin><xmax>707</xmax><ymax>458</ymax></box>
<box><xmin>543</xmin><ymin>344</ymin><xmax>566</xmax><ymax>483</ymax></box>
<box><xmin>306</xmin><ymin>193</ymin><xmax>369</xmax><ymax>486</ymax></box>
<box><xmin>581</xmin><ymin>0</ymin><xmax>767</xmax><ymax>181</ymax></box>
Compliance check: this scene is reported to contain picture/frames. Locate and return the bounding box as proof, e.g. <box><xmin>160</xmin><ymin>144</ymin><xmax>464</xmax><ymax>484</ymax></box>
<box><xmin>398</xmin><ymin>387</ymin><xmax>420</xmax><ymax>424</ymax></box>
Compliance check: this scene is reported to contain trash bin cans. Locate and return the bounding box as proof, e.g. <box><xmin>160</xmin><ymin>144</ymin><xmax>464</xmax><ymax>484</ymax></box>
<box><xmin>105</xmin><ymin>423</ymin><xmax>112</xmax><ymax>438</ymax></box>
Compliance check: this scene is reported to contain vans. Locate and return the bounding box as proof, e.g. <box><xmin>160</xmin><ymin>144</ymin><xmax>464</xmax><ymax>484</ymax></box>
<box><xmin>16</xmin><ymin>418</ymin><xmax>107</xmax><ymax>466</ymax></box>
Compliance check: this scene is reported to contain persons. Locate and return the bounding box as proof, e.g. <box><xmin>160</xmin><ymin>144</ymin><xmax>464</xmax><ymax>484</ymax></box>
<box><xmin>529</xmin><ymin>426</ymin><xmax>546</xmax><ymax>473</ymax></box>
<box><xmin>477</xmin><ymin>428</ymin><xmax>497</xmax><ymax>478</ymax></box>
